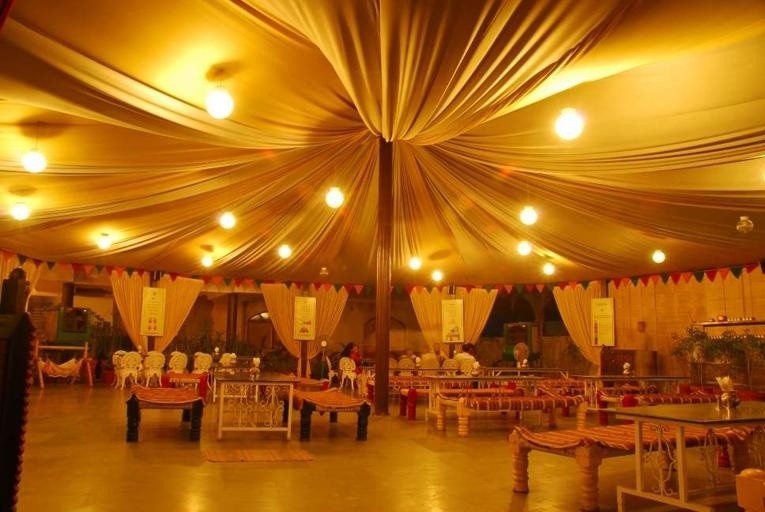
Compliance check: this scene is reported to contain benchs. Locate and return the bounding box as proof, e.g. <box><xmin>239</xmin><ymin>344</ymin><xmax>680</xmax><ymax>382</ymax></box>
<box><xmin>508</xmin><ymin>421</ymin><xmax>765</xmax><ymax>512</ymax></box>
<box><xmin>354</xmin><ymin>367</ymin><xmax>720</xmax><ymax>436</ymax></box>
<box><xmin>283</xmin><ymin>388</ymin><xmax>372</xmax><ymax>441</ymax></box>
<box><xmin>125</xmin><ymin>384</ymin><xmax>206</xmax><ymax>442</ymax></box>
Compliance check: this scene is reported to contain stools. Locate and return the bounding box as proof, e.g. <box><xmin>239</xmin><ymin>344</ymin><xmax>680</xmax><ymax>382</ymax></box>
<box><xmin>161</xmin><ymin>373</ymin><xmax>208</xmax><ymax>405</ymax></box>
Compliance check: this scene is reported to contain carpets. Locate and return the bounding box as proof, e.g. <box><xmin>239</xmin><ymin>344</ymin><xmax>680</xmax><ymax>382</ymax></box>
<box><xmin>200</xmin><ymin>422</ymin><xmax>315</xmax><ymax>461</ymax></box>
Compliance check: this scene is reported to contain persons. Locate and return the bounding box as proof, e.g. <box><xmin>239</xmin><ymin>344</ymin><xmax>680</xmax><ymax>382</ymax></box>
<box><xmin>430</xmin><ymin>342</ymin><xmax>448</xmax><ymax>366</ymax></box>
<box><xmin>398</xmin><ymin>349</ymin><xmax>416</xmax><ymax>359</ymax></box>
<box><xmin>452</xmin><ymin>343</ymin><xmax>475</xmax><ymax>368</ymax></box>
<box><xmin>339</xmin><ymin>341</ymin><xmax>358</xmax><ymax>370</ymax></box>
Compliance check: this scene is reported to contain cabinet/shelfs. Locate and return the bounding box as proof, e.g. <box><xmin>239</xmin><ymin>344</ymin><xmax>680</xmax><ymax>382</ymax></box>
<box><xmin>689</xmin><ymin>320</ymin><xmax>765</xmax><ymax>392</ymax></box>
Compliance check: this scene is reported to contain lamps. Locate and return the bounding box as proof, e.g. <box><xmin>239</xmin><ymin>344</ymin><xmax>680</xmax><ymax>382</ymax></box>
<box><xmin>205</xmin><ymin>68</ymin><xmax>235</xmax><ymax>120</ymax></box>
<box><xmin>554</xmin><ymin>88</ymin><xmax>584</xmax><ymax>140</ymax></box>
<box><xmin>407</xmin><ymin>173</ymin><xmax>666</xmax><ymax>283</ymax></box>
<box><xmin>735</xmin><ymin>216</ymin><xmax>754</xmax><ymax>234</ymax></box>
<box><xmin>319</xmin><ymin>266</ymin><xmax>329</xmax><ymax>277</ymax></box>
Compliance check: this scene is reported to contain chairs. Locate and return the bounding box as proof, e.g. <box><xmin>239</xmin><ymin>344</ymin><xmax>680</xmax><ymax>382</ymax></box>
<box><xmin>325</xmin><ymin>356</ymin><xmax>338</xmax><ymax>388</ymax></box>
<box><xmin>338</xmin><ymin>356</ymin><xmax>357</xmax><ymax>390</ymax></box>
<box><xmin>110</xmin><ymin>350</ymin><xmax>261</xmax><ymax>402</ymax></box>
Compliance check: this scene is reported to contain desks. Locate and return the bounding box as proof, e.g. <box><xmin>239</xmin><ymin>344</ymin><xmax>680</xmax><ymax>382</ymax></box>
<box><xmin>616</xmin><ymin>402</ymin><xmax>765</xmax><ymax>512</ymax></box>
<box><xmin>214</xmin><ymin>372</ymin><xmax>301</xmax><ymax>440</ymax></box>
